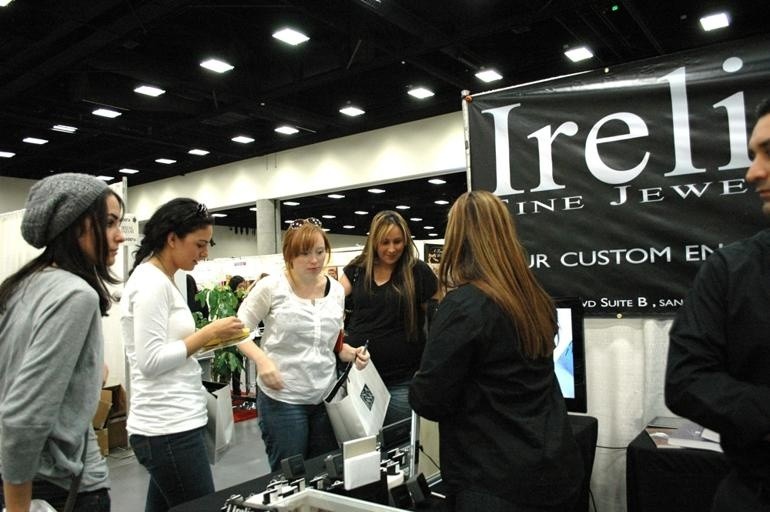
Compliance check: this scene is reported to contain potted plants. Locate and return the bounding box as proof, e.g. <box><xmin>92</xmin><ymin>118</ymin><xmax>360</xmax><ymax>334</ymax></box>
<box><xmin>192</xmin><ymin>275</ymin><xmax>267</xmax><ymax>421</ymax></box>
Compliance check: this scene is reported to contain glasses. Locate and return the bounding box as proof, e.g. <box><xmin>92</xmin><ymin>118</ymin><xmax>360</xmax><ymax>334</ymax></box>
<box><xmin>285</xmin><ymin>216</ymin><xmax>322</xmax><ymax>237</ymax></box>
<box><xmin>179</xmin><ymin>198</ymin><xmax>208</xmax><ymax>226</ymax></box>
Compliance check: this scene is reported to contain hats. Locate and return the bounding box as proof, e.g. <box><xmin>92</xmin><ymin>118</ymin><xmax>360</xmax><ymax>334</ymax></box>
<box><xmin>18</xmin><ymin>171</ymin><xmax>109</xmax><ymax>249</ymax></box>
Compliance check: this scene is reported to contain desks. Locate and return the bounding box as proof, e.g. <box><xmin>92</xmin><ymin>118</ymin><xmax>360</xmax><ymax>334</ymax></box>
<box><xmin>169</xmin><ymin>434</ymin><xmax>452</xmax><ymax>512</ymax></box>
<box><xmin>627</xmin><ymin>417</ymin><xmax>724</xmax><ymax>512</ymax></box>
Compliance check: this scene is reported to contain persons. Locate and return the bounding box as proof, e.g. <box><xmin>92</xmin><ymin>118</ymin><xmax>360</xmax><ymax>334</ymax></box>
<box><xmin>339</xmin><ymin>210</ymin><xmax>444</xmax><ymax>446</ymax></box>
<box><xmin>227</xmin><ymin>276</ymin><xmax>244</xmax><ymax>396</ymax></box>
<box><xmin>408</xmin><ymin>190</ymin><xmax>587</xmax><ymax>512</ymax></box>
<box><xmin>0</xmin><ymin>172</ymin><xmax>125</xmax><ymax>512</ymax></box>
<box><xmin>326</xmin><ymin>269</ymin><xmax>338</xmax><ymax>280</ymax></box>
<box><xmin>664</xmin><ymin>95</ymin><xmax>770</xmax><ymax>511</ymax></box>
<box><xmin>235</xmin><ymin>217</ymin><xmax>370</xmax><ymax>474</ymax></box>
<box><xmin>119</xmin><ymin>199</ymin><xmax>245</xmax><ymax>512</ymax></box>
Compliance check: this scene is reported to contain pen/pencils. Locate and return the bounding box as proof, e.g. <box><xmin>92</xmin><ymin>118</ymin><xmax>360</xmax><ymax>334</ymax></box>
<box><xmin>362</xmin><ymin>340</ymin><xmax>369</xmax><ymax>355</ymax></box>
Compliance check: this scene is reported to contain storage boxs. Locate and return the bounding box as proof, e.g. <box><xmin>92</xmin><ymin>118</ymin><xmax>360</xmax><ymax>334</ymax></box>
<box><xmin>102</xmin><ymin>384</ymin><xmax>127</xmax><ymax>418</ymax></box>
<box><xmin>106</xmin><ymin>417</ymin><xmax>128</xmax><ymax>452</ymax></box>
<box><xmin>96</xmin><ymin>429</ymin><xmax>109</xmax><ymax>457</ymax></box>
<box><xmin>93</xmin><ymin>390</ymin><xmax>113</xmax><ymax>428</ymax></box>
<box><xmin>103</xmin><ymin>364</ymin><xmax>108</xmax><ymax>386</ymax></box>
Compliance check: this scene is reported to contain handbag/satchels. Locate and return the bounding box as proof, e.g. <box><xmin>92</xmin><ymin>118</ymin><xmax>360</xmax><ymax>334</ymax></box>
<box><xmin>321</xmin><ymin>341</ymin><xmax>393</xmax><ymax>451</ymax></box>
<box><xmin>194</xmin><ymin>368</ymin><xmax>238</xmax><ymax>462</ymax></box>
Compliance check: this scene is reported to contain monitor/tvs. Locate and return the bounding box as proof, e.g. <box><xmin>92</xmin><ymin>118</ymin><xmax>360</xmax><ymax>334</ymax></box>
<box><xmin>410</xmin><ymin>410</ymin><xmax>443</xmax><ymax>487</ymax></box>
<box><xmin>554</xmin><ymin>297</ymin><xmax>587</xmax><ymax>413</ymax></box>
